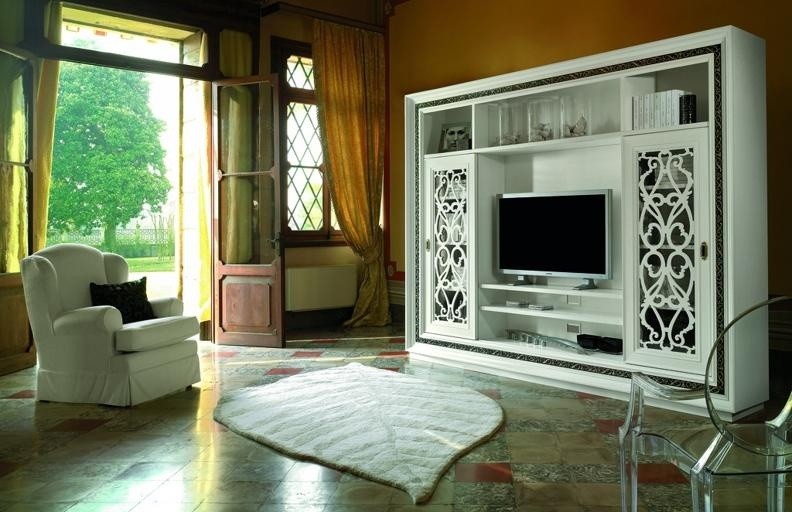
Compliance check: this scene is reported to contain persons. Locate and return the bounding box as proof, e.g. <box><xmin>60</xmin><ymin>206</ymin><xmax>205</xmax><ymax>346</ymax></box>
<box><xmin>446</xmin><ymin>126</ymin><xmax>466</xmax><ymax>151</ymax></box>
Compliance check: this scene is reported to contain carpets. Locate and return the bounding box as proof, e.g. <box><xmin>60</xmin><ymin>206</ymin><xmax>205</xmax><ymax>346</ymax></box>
<box><xmin>212</xmin><ymin>361</ymin><xmax>505</xmax><ymax>504</ymax></box>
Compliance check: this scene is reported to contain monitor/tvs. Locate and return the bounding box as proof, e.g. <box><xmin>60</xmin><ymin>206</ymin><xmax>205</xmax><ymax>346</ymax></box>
<box><xmin>493</xmin><ymin>186</ymin><xmax>613</xmax><ymax>290</ymax></box>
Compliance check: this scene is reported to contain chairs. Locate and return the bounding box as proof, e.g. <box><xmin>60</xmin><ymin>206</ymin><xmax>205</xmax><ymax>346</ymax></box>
<box><xmin>22</xmin><ymin>243</ymin><xmax>200</xmax><ymax>410</ymax></box>
<box><xmin>616</xmin><ymin>295</ymin><xmax>792</xmax><ymax>512</ymax></box>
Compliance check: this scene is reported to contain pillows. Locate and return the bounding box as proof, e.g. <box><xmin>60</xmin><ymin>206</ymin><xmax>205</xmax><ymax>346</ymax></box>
<box><xmin>89</xmin><ymin>275</ymin><xmax>157</xmax><ymax>326</ymax></box>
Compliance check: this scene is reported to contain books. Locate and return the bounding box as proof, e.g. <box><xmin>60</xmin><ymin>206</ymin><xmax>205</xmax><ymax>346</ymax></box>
<box><xmin>506</xmin><ymin>300</ymin><xmax>553</xmax><ymax>311</ymax></box>
<box><xmin>632</xmin><ymin>90</ymin><xmax>696</xmax><ymax>183</ymax></box>
<box><xmin>439</xmin><ymin>179</ymin><xmax>467</xmax><ymax>287</ymax></box>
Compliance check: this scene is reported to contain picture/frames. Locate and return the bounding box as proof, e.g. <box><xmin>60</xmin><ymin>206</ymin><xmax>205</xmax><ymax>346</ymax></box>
<box><xmin>438</xmin><ymin>122</ymin><xmax>472</xmax><ymax>152</ymax></box>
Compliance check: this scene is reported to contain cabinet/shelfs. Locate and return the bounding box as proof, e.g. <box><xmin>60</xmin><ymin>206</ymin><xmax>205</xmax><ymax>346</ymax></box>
<box><xmin>404</xmin><ymin>23</ymin><xmax>772</xmax><ymax>423</ymax></box>
